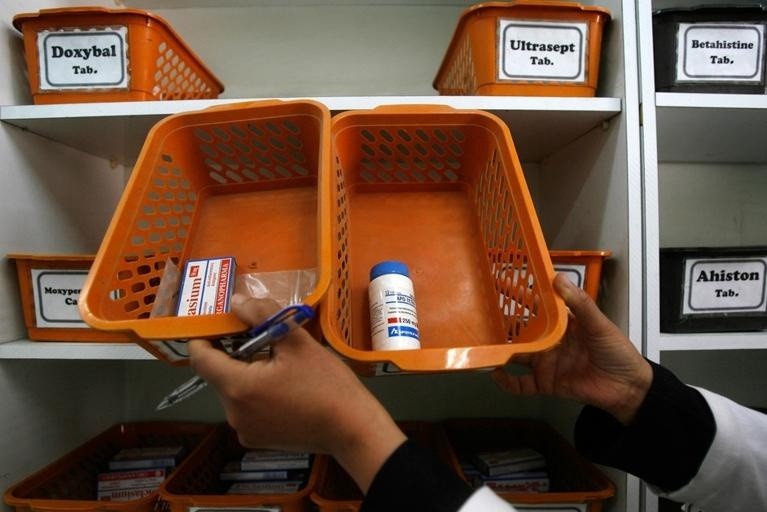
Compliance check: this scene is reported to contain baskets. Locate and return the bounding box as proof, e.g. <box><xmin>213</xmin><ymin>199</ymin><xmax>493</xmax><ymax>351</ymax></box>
<box><xmin>311</xmin><ymin>420</ymin><xmax>424</xmax><ymax>512</ymax></box>
<box><xmin>156</xmin><ymin>421</ymin><xmax>318</xmax><ymax>512</ymax></box>
<box><xmin>430</xmin><ymin>0</ymin><xmax>615</xmax><ymax>97</ymax></box>
<box><xmin>72</xmin><ymin>97</ymin><xmax>336</xmax><ymax>343</ymax></box>
<box><xmin>316</xmin><ymin>102</ymin><xmax>572</xmax><ymax>378</ymax></box>
<box><xmin>649</xmin><ymin>0</ymin><xmax>766</xmax><ymax>97</ymax></box>
<box><xmin>0</xmin><ymin>411</ymin><xmax>622</xmax><ymax>512</ymax></box>
<box><xmin>4</xmin><ymin>421</ymin><xmax>217</xmax><ymax>512</ymax></box>
<box><xmin>443</xmin><ymin>414</ymin><xmax>619</xmax><ymax>512</ymax></box>
<box><xmin>7</xmin><ymin>4</ymin><xmax>228</xmax><ymax>108</ymax></box>
<box><xmin>656</xmin><ymin>244</ymin><xmax>767</xmax><ymax>336</ymax></box>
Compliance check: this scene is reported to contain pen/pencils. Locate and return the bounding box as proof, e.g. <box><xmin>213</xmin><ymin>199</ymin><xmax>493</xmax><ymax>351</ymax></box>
<box><xmin>155</xmin><ymin>303</ymin><xmax>314</xmax><ymax>412</ymax></box>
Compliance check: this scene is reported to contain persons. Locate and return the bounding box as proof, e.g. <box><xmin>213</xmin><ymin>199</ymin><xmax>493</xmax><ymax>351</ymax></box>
<box><xmin>185</xmin><ymin>269</ymin><xmax>766</xmax><ymax>511</ymax></box>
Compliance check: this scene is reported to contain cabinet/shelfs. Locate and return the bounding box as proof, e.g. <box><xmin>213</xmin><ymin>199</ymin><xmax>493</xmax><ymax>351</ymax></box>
<box><xmin>1</xmin><ymin>1</ymin><xmax>647</xmax><ymax>510</ymax></box>
<box><xmin>631</xmin><ymin>0</ymin><xmax>765</xmax><ymax>511</ymax></box>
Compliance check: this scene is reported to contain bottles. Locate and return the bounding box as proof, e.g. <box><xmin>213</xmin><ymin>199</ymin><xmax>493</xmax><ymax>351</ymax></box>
<box><xmin>367</xmin><ymin>260</ymin><xmax>420</xmax><ymax>350</ymax></box>
